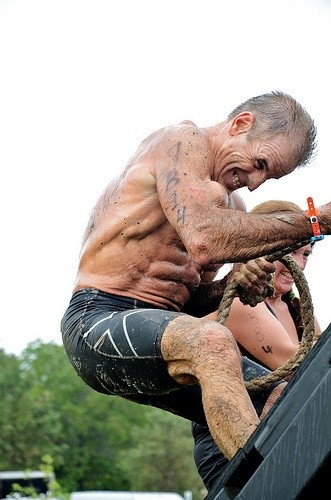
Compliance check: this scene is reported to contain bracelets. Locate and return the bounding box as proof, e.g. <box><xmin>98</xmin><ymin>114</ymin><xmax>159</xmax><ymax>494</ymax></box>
<box><xmin>305</xmin><ymin>196</ymin><xmax>324</xmax><ymax>245</ymax></box>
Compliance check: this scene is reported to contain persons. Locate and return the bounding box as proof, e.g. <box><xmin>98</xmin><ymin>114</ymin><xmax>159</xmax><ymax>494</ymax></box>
<box><xmin>186</xmin><ymin>198</ymin><xmax>321</xmax><ymax>498</ymax></box>
<box><xmin>57</xmin><ymin>88</ymin><xmax>331</xmax><ymax>468</ymax></box>
